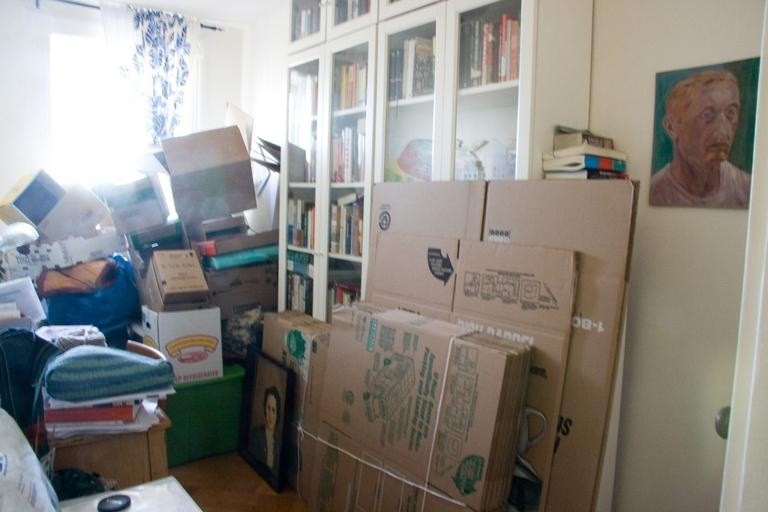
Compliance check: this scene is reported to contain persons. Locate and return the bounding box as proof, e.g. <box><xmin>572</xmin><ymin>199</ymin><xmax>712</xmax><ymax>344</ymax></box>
<box><xmin>250</xmin><ymin>386</ymin><xmax>281</xmax><ymax>470</ymax></box>
<box><xmin>648</xmin><ymin>68</ymin><xmax>750</xmax><ymax>207</ymax></box>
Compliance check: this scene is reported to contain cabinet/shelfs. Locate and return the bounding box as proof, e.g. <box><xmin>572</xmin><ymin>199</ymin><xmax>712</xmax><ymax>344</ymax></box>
<box><xmin>375</xmin><ymin>2</ymin><xmax>591</xmax><ymax>185</ymax></box>
<box><xmin>281</xmin><ymin>0</ymin><xmax>378</xmax><ymax>322</ymax></box>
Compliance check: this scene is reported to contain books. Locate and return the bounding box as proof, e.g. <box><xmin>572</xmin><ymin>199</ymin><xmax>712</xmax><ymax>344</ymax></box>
<box><xmin>329</xmin><ymin>191</ymin><xmax>364</xmax><ymax>257</ymax></box>
<box><xmin>327</xmin><ymin>258</ymin><xmax>361</xmax><ymax>324</ymax></box>
<box><xmin>291</xmin><ymin>1</ymin><xmax>370</xmax><ymax>41</ymax></box>
<box><xmin>539</xmin><ymin>125</ymin><xmax>630</xmax><ymax>180</ymax></box>
<box><xmin>286</xmin><ymin>249</ymin><xmax>314</xmax><ymax>317</ymax></box>
<box><xmin>287</xmin><ymin>196</ymin><xmax>315</xmax><ymax>249</ymax></box>
<box><xmin>42</xmin><ymin>384</ymin><xmax>178</xmax><ymax>439</ymax></box>
<box><xmin>288</xmin><ymin>57</ymin><xmax>368</xmax><ymax>183</ymax></box>
<box><xmin>389</xmin><ymin>11</ymin><xmax>519</xmax><ymax>101</ymax></box>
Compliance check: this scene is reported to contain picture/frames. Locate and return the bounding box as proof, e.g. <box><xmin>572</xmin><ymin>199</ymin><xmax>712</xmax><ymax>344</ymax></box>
<box><xmin>238</xmin><ymin>344</ymin><xmax>295</xmax><ymax>493</ymax></box>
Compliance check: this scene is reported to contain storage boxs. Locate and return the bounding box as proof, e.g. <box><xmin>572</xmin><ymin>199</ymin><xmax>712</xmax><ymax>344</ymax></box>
<box><xmin>40</xmin><ymin>169</ymin><xmax>107</xmax><ymax>241</ymax></box>
<box><xmin>106</xmin><ymin>173</ymin><xmax>167</xmax><ymax>235</ymax></box>
<box><xmin>150</xmin><ymin>126</ymin><xmax>257</xmax><ymax>221</ymax></box>
<box><xmin>1</xmin><ymin>166</ymin><xmax>65</xmax><ymax>226</ymax></box>
<box><xmin>144</xmin><ymin>249</ymin><xmax>209</xmax><ymax>312</ymax></box>
<box><xmin>140</xmin><ymin>304</ymin><xmax>224</xmax><ymax>384</ymax></box>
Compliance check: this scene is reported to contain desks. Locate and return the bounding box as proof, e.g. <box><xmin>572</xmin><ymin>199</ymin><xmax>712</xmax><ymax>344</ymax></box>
<box><xmin>42</xmin><ymin>386</ymin><xmax>172</xmax><ymax>490</ymax></box>
<box><xmin>59</xmin><ymin>475</ymin><xmax>204</xmax><ymax>512</ymax></box>
